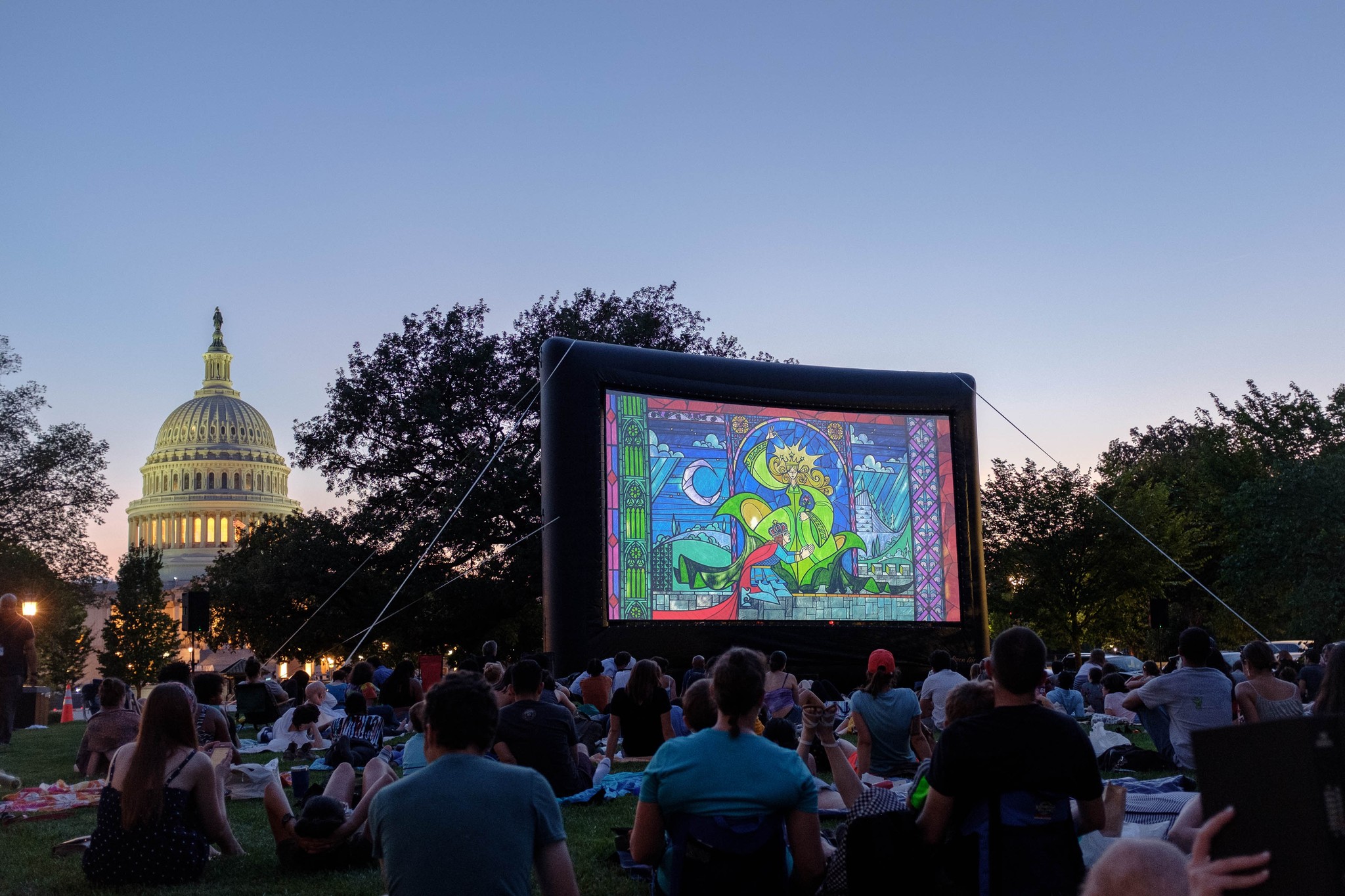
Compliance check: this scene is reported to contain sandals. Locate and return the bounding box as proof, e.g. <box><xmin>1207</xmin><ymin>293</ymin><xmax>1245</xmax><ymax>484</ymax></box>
<box><xmin>814</xmin><ymin>703</ymin><xmax>839</xmax><ymax>747</ymax></box>
<box><xmin>797</xmin><ymin>703</ymin><xmax>824</xmax><ymax>746</ymax></box>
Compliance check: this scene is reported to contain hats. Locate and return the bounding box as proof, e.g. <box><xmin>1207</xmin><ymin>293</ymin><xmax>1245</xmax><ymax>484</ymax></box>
<box><xmin>868</xmin><ymin>649</ymin><xmax>896</xmax><ymax>674</ymax></box>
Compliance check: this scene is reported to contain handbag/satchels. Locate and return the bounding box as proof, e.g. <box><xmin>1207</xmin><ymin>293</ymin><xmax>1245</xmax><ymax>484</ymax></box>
<box><xmin>1089</xmin><ymin>722</ymin><xmax>1132</xmax><ymax>758</ymax></box>
<box><xmin>53</xmin><ymin>835</ymin><xmax>91</xmax><ymax>857</ymax></box>
<box><xmin>1098</xmin><ymin>742</ymin><xmax>1163</xmax><ymax>772</ymax></box>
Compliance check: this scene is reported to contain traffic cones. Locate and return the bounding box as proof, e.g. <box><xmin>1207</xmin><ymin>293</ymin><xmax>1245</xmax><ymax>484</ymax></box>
<box><xmin>61</xmin><ymin>684</ymin><xmax>74</xmax><ymax>722</ymax></box>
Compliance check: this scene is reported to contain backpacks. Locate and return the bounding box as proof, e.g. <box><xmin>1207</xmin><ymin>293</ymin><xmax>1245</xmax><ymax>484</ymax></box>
<box><xmin>572</xmin><ymin>709</ymin><xmax>605</xmax><ymax>756</ymax></box>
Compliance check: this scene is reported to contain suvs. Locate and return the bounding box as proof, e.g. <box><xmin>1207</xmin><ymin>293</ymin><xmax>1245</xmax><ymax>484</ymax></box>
<box><xmin>1265</xmin><ymin>640</ymin><xmax>1324</xmax><ymax>665</ymax></box>
<box><xmin>1062</xmin><ymin>652</ymin><xmax>1144</xmax><ymax>682</ymax></box>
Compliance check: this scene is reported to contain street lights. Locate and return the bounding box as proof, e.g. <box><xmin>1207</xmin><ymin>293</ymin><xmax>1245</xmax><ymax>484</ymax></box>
<box><xmin>188</xmin><ymin>645</ymin><xmax>193</xmax><ymax>661</ymax></box>
<box><xmin>21</xmin><ymin>593</ymin><xmax>38</xmax><ymax>621</ymax></box>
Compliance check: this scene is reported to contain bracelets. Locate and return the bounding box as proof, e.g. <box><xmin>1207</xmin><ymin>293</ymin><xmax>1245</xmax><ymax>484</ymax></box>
<box><xmin>31</xmin><ymin>671</ymin><xmax>38</xmax><ymax>675</ymax></box>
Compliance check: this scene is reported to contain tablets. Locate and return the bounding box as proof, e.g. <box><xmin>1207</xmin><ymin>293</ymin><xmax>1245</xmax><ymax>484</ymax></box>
<box><xmin>1192</xmin><ymin>716</ymin><xmax>1335</xmax><ymax>896</ymax></box>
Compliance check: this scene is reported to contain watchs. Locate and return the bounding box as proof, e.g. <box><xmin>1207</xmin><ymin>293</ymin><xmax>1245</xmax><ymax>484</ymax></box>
<box><xmin>281</xmin><ymin>812</ymin><xmax>296</xmax><ymax>829</ymax></box>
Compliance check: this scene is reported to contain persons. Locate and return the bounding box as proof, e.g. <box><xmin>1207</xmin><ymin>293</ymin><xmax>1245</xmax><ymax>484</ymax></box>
<box><xmin>914</xmin><ymin>627</ymin><xmax>1107</xmax><ymax>896</ymax></box>
<box><xmin>367</xmin><ymin>669</ymin><xmax>581</xmax><ymax>896</ymax></box>
<box><xmin>629</xmin><ymin>646</ymin><xmax>826</xmax><ymax>896</ymax></box>
<box><xmin>73</xmin><ymin>626</ymin><xmax>1345</xmax><ymax>896</ymax></box>
<box><xmin>0</xmin><ymin>594</ymin><xmax>39</xmax><ymax>745</ymax></box>
<box><xmin>80</xmin><ymin>681</ymin><xmax>249</xmax><ymax>885</ymax></box>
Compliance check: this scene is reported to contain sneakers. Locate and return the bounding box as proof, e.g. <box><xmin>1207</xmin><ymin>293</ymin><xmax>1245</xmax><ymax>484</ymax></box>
<box><xmin>295</xmin><ymin>742</ymin><xmax>321</xmax><ymax>763</ymax></box>
<box><xmin>281</xmin><ymin>741</ymin><xmax>299</xmax><ymax>762</ymax></box>
<box><xmin>257</xmin><ymin>726</ymin><xmax>273</xmax><ymax>743</ymax></box>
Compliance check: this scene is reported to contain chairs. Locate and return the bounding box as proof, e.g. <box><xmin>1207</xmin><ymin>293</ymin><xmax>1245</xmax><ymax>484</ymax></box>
<box><xmin>614</xmin><ymin>809</ymin><xmax>790</xmax><ymax>896</ymax></box>
<box><xmin>82</xmin><ymin>678</ymin><xmax>141</xmax><ymax>722</ymax></box>
<box><xmin>233</xmin><ymin>683</ymin><xmax>296</xmax><ymax>735</ymax></box>
<box><xmin>821</xmin><ymin>809</ymin><xmax>980</xmax><ymax>896</ymax></box>
